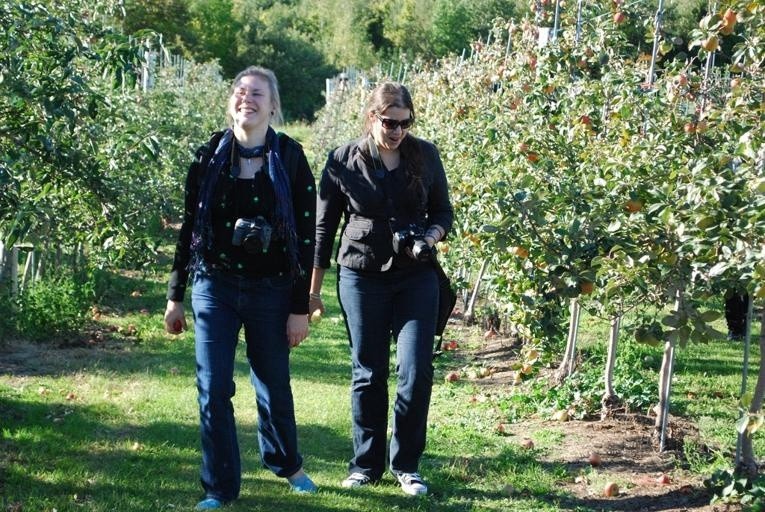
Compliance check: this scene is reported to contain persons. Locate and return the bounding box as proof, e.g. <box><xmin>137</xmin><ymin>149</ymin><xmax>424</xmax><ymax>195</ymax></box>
<box><xmin>308</xmin><ymin>83</ymin><xmax>455</xmax><ymax>497</ymax></box>
<box><xmin>164</xmin><ymin>64</ymin><xmax>319</xmax><ymax>510</ymax></box>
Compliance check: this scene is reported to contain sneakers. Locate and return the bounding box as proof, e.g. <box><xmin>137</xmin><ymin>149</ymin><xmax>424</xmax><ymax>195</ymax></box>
<box><xmin>390</xmin><ymin>467</ymin><xmax>429</xmax><ymax>497</ymax></box>
<box><xmin>344</xmin><ymin>473</ymin><xmax>373</xmax><ymax>488</ymax></box>
<box><xmin>197</xmin><ymin>499</ymin><xmax>223</xmax><ymax>512</ymax></box>
<box><xmin>288</xmin><ymin>472</ymin><xmax>319</xmax><ymax>493</ymax></box>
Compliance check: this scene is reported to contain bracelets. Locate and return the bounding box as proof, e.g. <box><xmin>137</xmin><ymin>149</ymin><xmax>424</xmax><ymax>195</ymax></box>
<box><xmin>309</xmin><ymin>292</ymin><xmax>322</xmax><ymax>299</ymax></box>
<box><xmin>424</xmin><ymin>226</ymin><xmax>442</xmax><ymax>244</ymax></box>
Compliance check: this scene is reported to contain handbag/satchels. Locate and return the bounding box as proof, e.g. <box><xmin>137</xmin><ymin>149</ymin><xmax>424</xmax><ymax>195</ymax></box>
<box><xmin>436</xmin><ymin>283</ymin><xmax>457</xmax><ymax>335</ymax></box>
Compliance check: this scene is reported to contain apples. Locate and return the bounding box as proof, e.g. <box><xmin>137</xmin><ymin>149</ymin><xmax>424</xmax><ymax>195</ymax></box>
<box><xmin>56</xmin><ymin>290</ymin><xmax>148</xmax><ymax>346</ymax></box>
<box><xmin>438</xmin><ymin>0</ymin><xmax>765</xmax><ymax>497</ymax></box>
<box><xmin>311</xmin><ymin>308</ymin><xmax>321</xmax><ymax>324</ymax></box>
<box><xmin>173</xmin><ymin>319</ymin><xmax>182</xmax><ymax>331</ymax></box>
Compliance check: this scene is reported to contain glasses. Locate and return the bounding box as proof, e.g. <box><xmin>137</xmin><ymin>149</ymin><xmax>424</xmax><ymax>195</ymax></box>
<box><xmin>375</xmin><ymin>112</ymin><xmax>416</xmax><ymax>129</ymax></box>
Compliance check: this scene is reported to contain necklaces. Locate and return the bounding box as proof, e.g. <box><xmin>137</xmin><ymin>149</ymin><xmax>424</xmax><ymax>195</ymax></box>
<box><xmin>247</xmin><ymin>158</ymin><xmax>252</xmax><ymax>165</ymax></box>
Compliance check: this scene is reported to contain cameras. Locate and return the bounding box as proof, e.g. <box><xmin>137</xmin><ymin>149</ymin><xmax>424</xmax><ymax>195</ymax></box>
<box><xmin>231</xmin><ymin>216</ymin><xmax>274</xmax><ymax>257</ymax></box>
<box><xmin>392</xmin><ymin>221</ymin><xmax>433</xmax><ymax>265</ymax></box>
